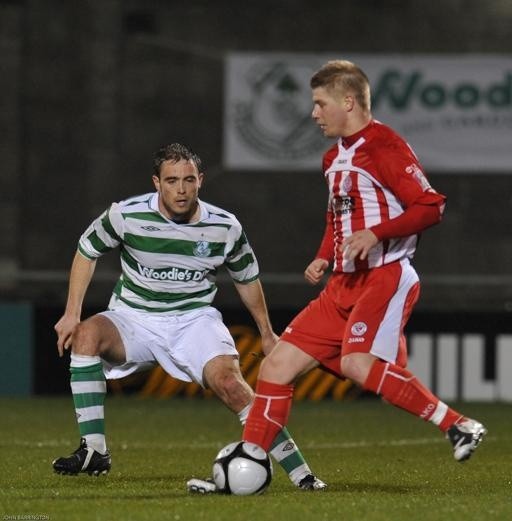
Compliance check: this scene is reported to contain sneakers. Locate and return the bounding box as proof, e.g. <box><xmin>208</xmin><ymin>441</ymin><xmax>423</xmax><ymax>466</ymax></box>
<box><xmin>295</xmin><ymin>471</ymin><xmax>328</xmax><ymax>491</ymax></box>
<box><xmin>186</xmin><ymin>476</ymin><xmax>216</xmax><ymax>493</ymax></box>
<box><xmin>444</xmin><ymin>417</ymin><xmax>488</xmax><ymax>462</ymax></box>
<box><xmin>52</xmin><ymin>437</ymin><xmax>112</xmax><ymax>476</ymax></box>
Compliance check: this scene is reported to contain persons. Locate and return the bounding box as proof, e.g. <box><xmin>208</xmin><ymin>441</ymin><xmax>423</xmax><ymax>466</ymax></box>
<box><xmin>50</xmin><ymin>141</ymin><xmax>334</xmax><ymax>497</ymax></box>
<box><xmin>180</xmin><ymin>56</ymin><xmax>488</xmax><ymax>497</ymax></box>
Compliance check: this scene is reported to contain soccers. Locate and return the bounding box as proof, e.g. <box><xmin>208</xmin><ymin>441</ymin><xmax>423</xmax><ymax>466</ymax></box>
<box><xmin>211</xmin><ymin>440</ymin><xmax>273</xmax><ymax>496</ymax></box>
